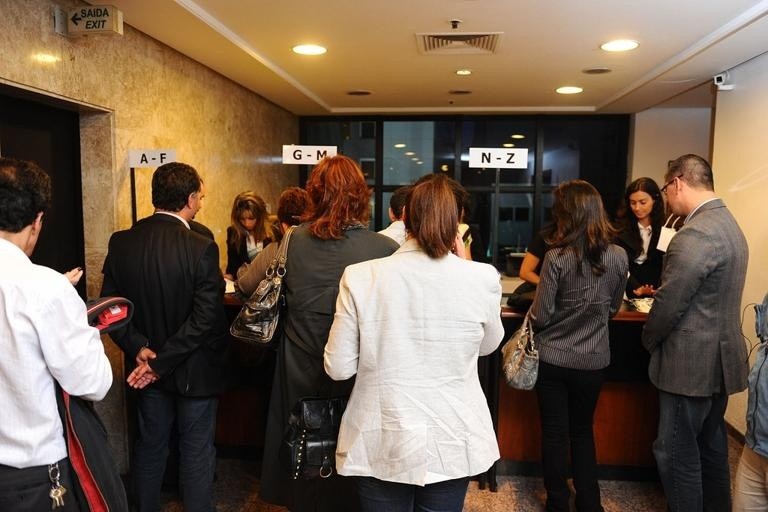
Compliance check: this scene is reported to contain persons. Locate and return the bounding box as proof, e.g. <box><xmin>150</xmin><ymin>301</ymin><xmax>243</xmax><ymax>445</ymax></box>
<box><xmin>59</xmin><ymin>260</ymin><xmax>84</xmax><ymax>285</ymax></box>
<box><xmin>528</xmin><ymin>180</ymin><xmax>630</xmax><ymax>511</ymax></box>
<box><xmin>732</xmin><ymin>292</ymin><xmax>768</xmax><ymax>512</ymax></box>
<box><xmin>323</xmin><ymin>173</ymin><xmax>506</xmax><ymax>511</ymax></box>
<box><xmin>100</xmin><ymin>162</ymin><xmax>229</xmax><ymax>511</ymax></box>
<box><xmin>640</xmin><ymin>153</ymin><xmax>749</xmax><ymax>511</ymax></box>
<box><xmin>102</xmin><ymin>178</ymin><xmax>678</xmax><ymax>302</ymax></box>
<box><xmin>0</xmin><ymin>157</ymin><xmax>114</xmax><ymax>512</ymax></box>
<box><xmin>258</xmin><ymin>155</ymin><xmax>401</xmax><ymax>511</ymax></box>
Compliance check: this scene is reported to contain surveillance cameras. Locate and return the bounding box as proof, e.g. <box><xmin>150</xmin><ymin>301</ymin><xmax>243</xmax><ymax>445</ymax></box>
<box><xmin>713</xmin><ymin>73</ymin><xmax>726</xmax><ymax>85</ymax></box>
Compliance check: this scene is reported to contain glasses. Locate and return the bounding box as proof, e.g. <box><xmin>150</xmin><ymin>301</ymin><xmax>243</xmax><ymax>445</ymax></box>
<box><xmin>659</xmin><ymin>174</ymin><xmax>684</xmax><ymax>193</ymax></box>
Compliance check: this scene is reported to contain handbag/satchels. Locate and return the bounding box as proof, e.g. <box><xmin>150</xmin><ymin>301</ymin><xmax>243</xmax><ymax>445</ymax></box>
<box><xmin>279</xmin><ymin>393</ymin><xmax>350</xmax><ymax>486</ymax></box>
<box><xmin>502</xmin><ymin>305</ymin><xmax>541</xmax><ymax>392</ymax></box>
<box><xmin>228</xmin><ymin>224</ymin><xmax>299</xmax><ymax>344</ymax></box>
